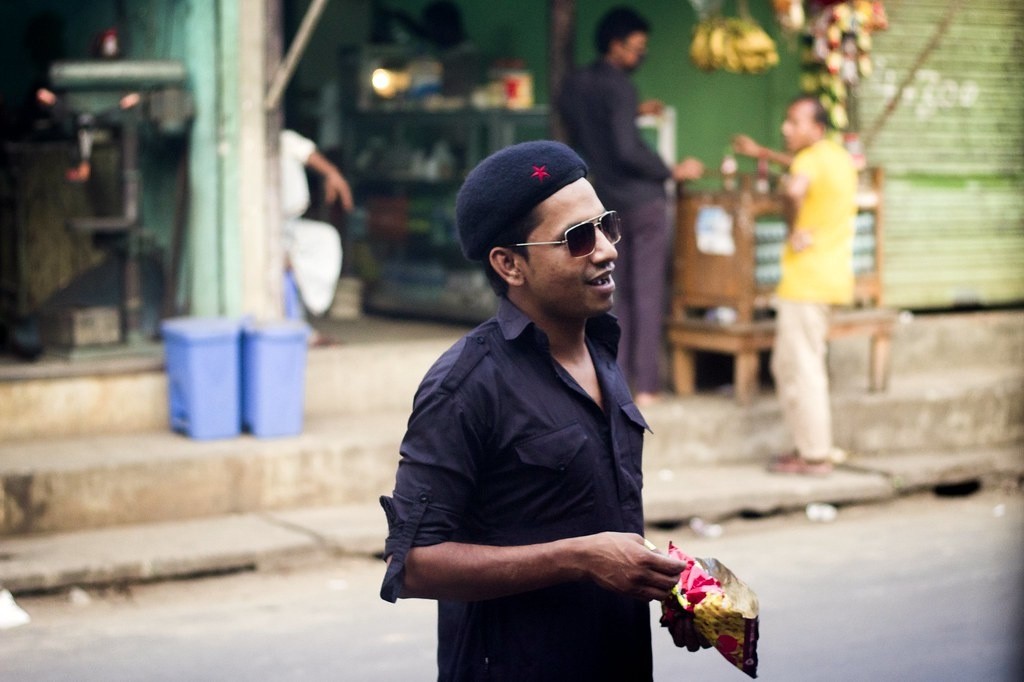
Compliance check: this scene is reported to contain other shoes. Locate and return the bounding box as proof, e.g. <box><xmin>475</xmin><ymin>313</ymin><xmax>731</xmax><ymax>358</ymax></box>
<box><xmin>770</xmin><ymin>448</ymin><xmax>832</xmax><ymax>476</ymax></box>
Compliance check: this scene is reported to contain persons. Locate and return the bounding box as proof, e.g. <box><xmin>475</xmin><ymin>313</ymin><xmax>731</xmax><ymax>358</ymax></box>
<box><xmin>733</xmin><ymin>89</ymin><xmax>859</xmax><ymax>480</ymax></box>
<box><xmin>378</xmin><ymin>141</ymin><xmax>712</xmax><ymax>682</ymax></box>
<box><xmin>276</xmin><ymin>126</ymin><xmax>355</xmax><ymax>218</ymax></box>
<box><xmin>559</xmin><ymin>8</ymin><xmax>708</xmax><ymax>409</ymax></box>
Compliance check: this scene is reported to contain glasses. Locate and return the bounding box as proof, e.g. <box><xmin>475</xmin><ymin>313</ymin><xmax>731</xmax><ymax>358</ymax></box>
<box><xmin>510</xmin><ymin>210</ymin><xmax>624</xmax><ymax>258</ymax></box>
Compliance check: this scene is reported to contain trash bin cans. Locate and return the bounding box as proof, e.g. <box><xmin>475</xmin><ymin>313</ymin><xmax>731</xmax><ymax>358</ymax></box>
<box><xmin>161</xmin><ymin>319</ymin><xmax>242</xmax><ymax>440</ymax></box>
<box><xmin>245</xmin><ymin>324</ymin><xmax>315</xmax><ymax>439</ymax></box>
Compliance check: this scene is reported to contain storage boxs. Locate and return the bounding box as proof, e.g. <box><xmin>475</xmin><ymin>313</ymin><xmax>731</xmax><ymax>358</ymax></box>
<box><xmin>44</xmin><ymin>305</ymin><xmax>122</xmax><ymax>346</ymax></box>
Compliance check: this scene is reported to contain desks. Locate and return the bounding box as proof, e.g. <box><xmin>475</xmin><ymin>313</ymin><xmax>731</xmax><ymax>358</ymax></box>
<box><xmin>667</xmin><ymin>309</ymin><xmax>902</xmax><ymax>404</ymax></box>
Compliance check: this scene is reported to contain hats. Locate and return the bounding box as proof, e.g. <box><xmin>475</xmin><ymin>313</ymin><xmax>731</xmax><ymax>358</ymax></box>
<box><xmin>456</xmin><ymin>139</ymin><xmax>588</xmax><ymax>260</ymax></box>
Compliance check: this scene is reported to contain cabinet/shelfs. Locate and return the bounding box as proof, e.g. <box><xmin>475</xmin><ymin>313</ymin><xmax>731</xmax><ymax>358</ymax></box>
<box><xmin>672</xmin><ymin>162</ymin><xmax>884</xmax><ymax>329</ymax></box>
<box><xmin>341</xmin><ymin>100</ymin><xmax>556</xmax><ymax>325</ymax></box>
